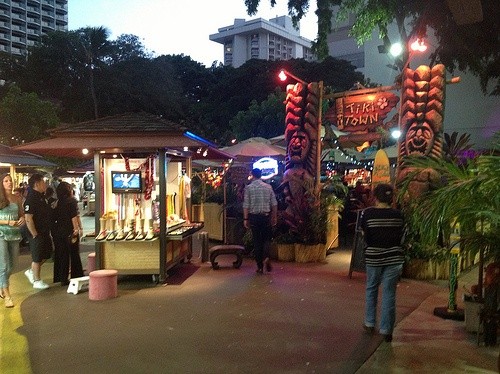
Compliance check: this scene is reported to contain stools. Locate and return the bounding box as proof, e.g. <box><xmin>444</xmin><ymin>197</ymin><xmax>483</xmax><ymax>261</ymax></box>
<box><xmin>208</xmin><ymin>243</ymin><xmax>246</xmax><ymax>271</ymax></box>
<box><xmin>88</xmin><ymin>252</ymin><xmax>95</xmax><ymax>274</ymax></box>
<box><xmin>67</xmin><ymin>275</ymin><xmax>90</xmax><ymax>295</ymax></box>
<box><xmin>86</xmin><ymin>269</ymin><xmax>120</xmax><ymax>299</ymax></box>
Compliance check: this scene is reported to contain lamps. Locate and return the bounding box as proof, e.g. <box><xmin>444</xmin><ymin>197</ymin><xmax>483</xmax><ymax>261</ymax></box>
<box><xmin>276</xmin><ymin>65</ymin><xmax>287</xmax><ymax>82</ymax></box>
<box><xmin>387</xmin><ymin>43</ymin><xmax>404</xmax><ymax>58</ymax></box>
<box><xmin>410</xmin><ymin>36</ymin><xmax>428</xmax><ymax>52</ymax></box>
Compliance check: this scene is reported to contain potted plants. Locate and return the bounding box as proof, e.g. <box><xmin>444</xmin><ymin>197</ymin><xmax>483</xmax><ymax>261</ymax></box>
<box><xmin>266</xmin><ymin>187</ymin><xmax>331</xmax><ymax>264</ymax></box>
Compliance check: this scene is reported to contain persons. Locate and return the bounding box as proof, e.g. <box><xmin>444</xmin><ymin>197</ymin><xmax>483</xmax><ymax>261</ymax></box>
<box><xmin>357</xmin><ymin>181</ymin><xmax>411</xmax><ymax>343</ymax></box>
<box><xmin>242</xmin><ymin>166</ymin><xmax>280</xmax><ymax>275</ymax></box>
<box><xmin>0</xmin><ymin>173</ymin><xmax>84</xmax><ymax>308</ymax></box>
<box><xmin>343</xmin><ymin>180</ymin><xmax>368</xmax><ymax>197</ymax></box>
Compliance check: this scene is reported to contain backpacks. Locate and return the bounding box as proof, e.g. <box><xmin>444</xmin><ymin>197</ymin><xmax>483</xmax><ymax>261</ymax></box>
<box><xmin>50</xmin><ymin>200</ymin><xmax>69</xmax><ymax>239</ymax></box>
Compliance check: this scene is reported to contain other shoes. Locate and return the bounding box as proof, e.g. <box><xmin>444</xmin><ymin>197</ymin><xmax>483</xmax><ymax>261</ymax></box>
<box><xmin>265</xmin><ymin>257</ymin><xmax>273</xmax><ymax>272</ymax></box>
<box><xmin>4</xmin><ymin>297</ymin><xmax>14</xmax><ymax>308</ymax></box>
<box><xmin>0</xmin><ymin>293</ymin><xmax>5</xmax><ymax>299</ymax></box>
<box><xmin>383</xmin><ymin>334</ymin><xmax>392</xmax><ymax>342</ymax></box>
<box><xmin>255</xmin><ymin>268</ymin><xmax>263</xmax><ymax>273</ymax></box>
<box><xmin>363</xmin><ymin>324</ymin><xmax>375</xmax><ymax>335</ymax></box>
<box><xmin>24</xmin><ymin>269</ymin><xmax>34</xmax><ymax>284</ymax></box>
<box><xmin>33</xmin><ymin>280</ymin><xmax>49</xmax><ymax>289</ymax></box>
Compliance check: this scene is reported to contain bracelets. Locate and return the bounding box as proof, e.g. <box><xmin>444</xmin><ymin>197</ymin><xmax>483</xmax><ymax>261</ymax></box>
<box><xmin>79</xmin><ymin>227</ymin><xmax>83</xmax><ymax>230</ymax></box>
<box><xmin>8</xmin><ymin>220</ymin><xmax>9</xmax><ymax>226</ymax></box>
<box><xmin>243</xmin><ymin>218</ymin><xmax>248</xmax><ymax>221</ymax></box>
